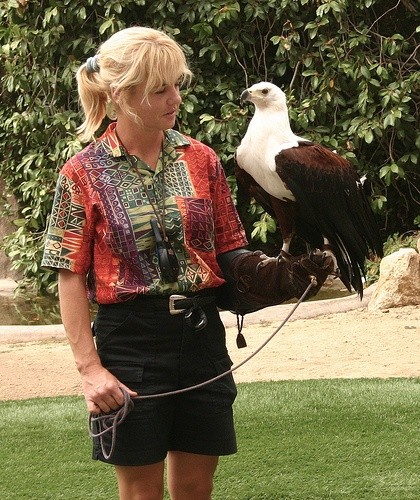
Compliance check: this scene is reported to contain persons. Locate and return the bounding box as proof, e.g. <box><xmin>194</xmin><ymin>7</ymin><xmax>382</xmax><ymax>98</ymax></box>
<box><xmin>40</xmin><ymin>26</ymin><xmax>329</xmax><ymax>500</ymax></box>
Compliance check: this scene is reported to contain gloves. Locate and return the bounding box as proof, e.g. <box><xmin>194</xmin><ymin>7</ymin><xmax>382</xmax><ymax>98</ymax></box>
<box><xmin>232</xmin><ymin>248</ymin><xmax>335</xmax><ymax>316</ymax></box>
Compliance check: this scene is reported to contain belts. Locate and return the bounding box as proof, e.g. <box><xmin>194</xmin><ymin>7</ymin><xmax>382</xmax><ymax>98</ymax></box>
<box><xmin>143</xmin><ymin>294</ymin><xmax>217</xmax><ymax>335</ymax></box>
